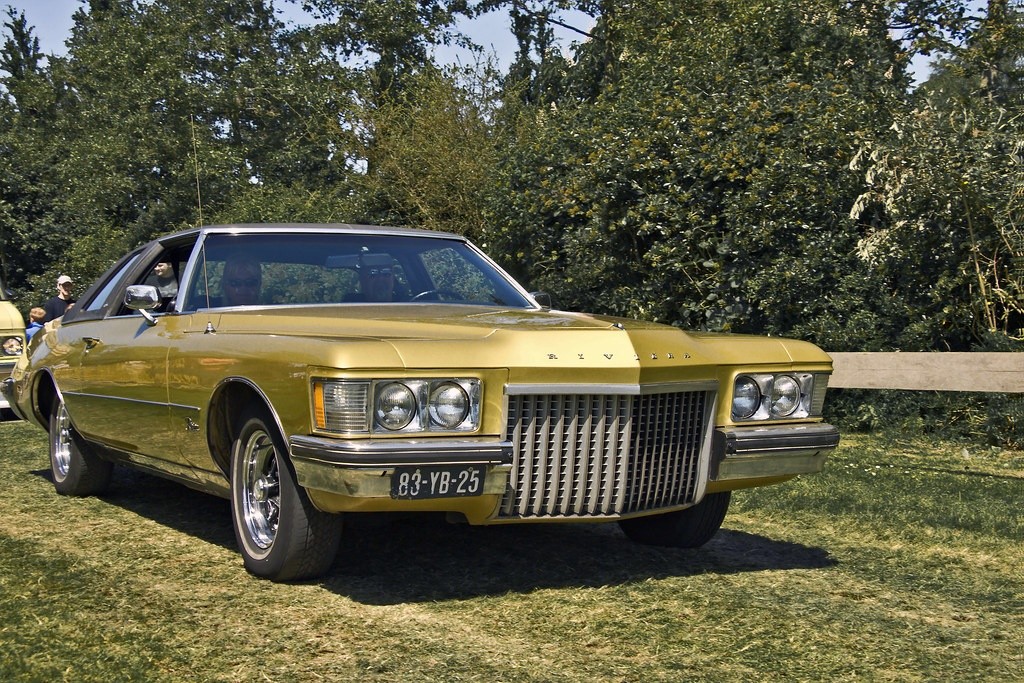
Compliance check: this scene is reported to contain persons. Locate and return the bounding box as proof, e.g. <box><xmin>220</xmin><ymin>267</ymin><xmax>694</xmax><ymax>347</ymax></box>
<box><xmin>151</xmin><ymin>262</ymin><xmax>178</xmax><ymax>297</ymax></box>
<box><xmin>220</xmin><ymin>252</ymin><xmax>263</xmax><ymax>306</ymax></box>
<box><xmin>342</xmin><ymin>265</ymin><xmax>409</xmax><ymax>302</ymax></box>
<box><xmin>44</xmin><ymin>275</ymin><xmax>77</xmax><ymax>325</ymax></box>
<box><xmin>25</xmin><ymin>307</ymin><xmax>46</xmax><ymax>345</ymax></box>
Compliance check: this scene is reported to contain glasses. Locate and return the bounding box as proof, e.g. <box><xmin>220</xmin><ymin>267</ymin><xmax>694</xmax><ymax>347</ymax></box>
<box><xmin>368</xmin><ymin>266</ymin><xmax>392</xmax><ymax>279</ymax></box>
<box><xmin>227</xmin><ymin>277</ymin><xmax>259</xmax><ymax>287</ymax></box>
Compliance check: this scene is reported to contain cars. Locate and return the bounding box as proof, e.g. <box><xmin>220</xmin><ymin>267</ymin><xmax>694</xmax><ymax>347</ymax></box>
<box><xmin>0</xmin><ymin>223</ymin><xmax>840</xmax><ymax>585</ymax></box>
<box><xmin>0</xmin><ymin>282</ymin><xmax>28</xmax><ymax>410</ymax></box>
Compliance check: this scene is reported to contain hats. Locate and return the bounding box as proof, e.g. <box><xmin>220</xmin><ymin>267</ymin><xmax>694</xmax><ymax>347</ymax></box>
<box><xmin>57</xmin><ymin>275</ymin><xmax>72</xmax><ymax>284</ymax></box>
